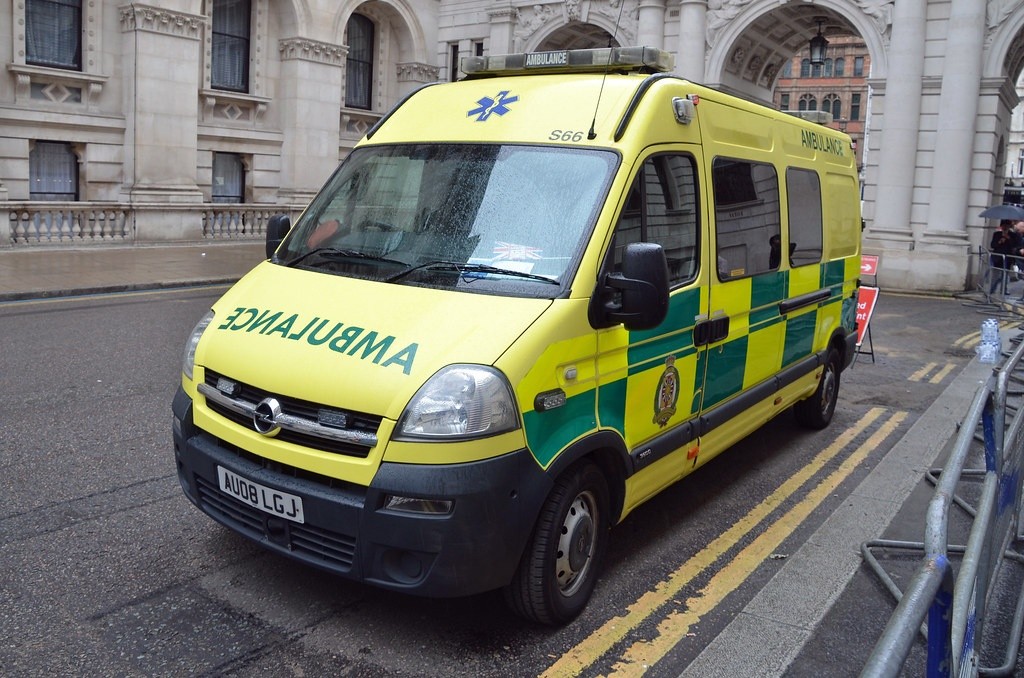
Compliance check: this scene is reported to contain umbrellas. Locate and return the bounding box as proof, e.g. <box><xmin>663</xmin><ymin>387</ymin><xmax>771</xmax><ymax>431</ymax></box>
<box><xmin>977</xmin><ymin>204</ymin><xmax>1024</xmax><ymax>246</ymax></box>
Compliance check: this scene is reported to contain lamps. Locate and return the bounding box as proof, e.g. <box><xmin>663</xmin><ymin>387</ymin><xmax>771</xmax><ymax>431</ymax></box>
<box><xmin>810</xmin><ymin>18</ymin><xmax>829</xmax><ymax>70</ymax></box>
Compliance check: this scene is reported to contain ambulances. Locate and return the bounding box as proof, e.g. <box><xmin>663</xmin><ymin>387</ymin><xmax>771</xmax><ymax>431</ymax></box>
<box><xmin>171</xmin><ymin>46</ymin><xmax>862</xmax><ymax>631</ymax></box>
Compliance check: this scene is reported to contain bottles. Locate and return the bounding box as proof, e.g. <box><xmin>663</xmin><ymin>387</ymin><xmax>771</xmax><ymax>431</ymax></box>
<box><xmin>978</xmin><ymin>319</ymin><xmax>1001</xmax><ymax>363</ymax></box>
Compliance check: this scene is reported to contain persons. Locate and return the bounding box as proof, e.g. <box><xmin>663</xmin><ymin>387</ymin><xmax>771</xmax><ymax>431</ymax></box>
<box><xmin>985</xmin><ymin>220</ymin><xmax>1023</xmax><ymax>296</ymax></box>
